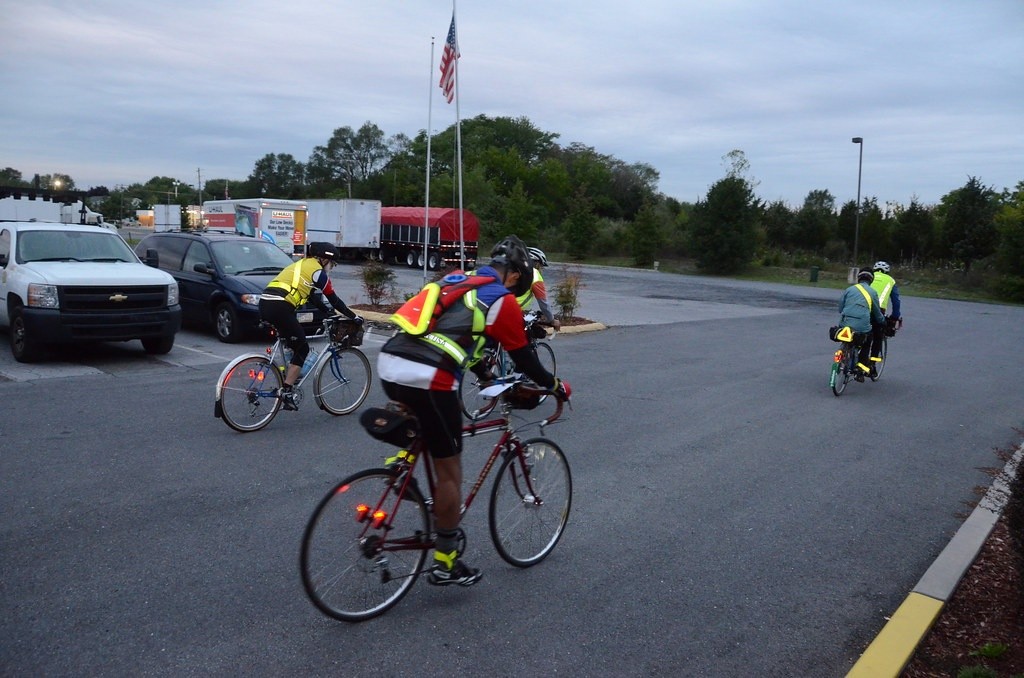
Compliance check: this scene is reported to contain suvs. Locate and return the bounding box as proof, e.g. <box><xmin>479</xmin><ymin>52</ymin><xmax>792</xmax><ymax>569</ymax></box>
<box><xmin>0</xmin><ymin>221</ymin><xmax>183</xmax><ymax>363</ymax></box>
<box><xmin>133</xmin><ymin>229</ymin><xmax>336</xmax><ymax>345</ymax></box>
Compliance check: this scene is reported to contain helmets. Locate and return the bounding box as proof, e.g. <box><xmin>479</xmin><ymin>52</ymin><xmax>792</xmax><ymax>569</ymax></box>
<box><xmin>310</xmin><ymin>241</ymin><xmax>341</xmax><ymax>262</ymax></box>
<box><xmin>858</xmin><ymin>267</ymin><xmax>874</xmax><ymax>285</ymax></box>
<box><xmin>526</xmin><ymin>246</ymin><xmax>550</xmax><ymax>265</ymax></box>
<box><xmin>490</xmin><ymin>234</ymin><xmax>533</xmax><ymax>297</ymax></box>
<box><xmin>874</xmin><ymin>261</ymin><xmax>890</xmax><ymax>273</ymax></box>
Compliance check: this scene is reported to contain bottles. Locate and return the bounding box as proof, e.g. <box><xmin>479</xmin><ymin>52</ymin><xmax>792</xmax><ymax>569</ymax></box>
<box><xmin>299</xmin><ymin>350</ymin><xmax>319</xmax><ymax>377</ymax></box>
<box><xmin>283</xmin><ymin>346</ymin><xmax>294</xmax><ymax>366</ymax></box>
<box><xmin>504</xmin><ymin>357</ymin><xmax>515</xmax><ymax>371</ymax></box>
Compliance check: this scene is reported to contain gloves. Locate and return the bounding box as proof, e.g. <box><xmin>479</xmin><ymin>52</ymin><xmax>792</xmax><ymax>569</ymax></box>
<box><xmin>553</xmin><ymin>380</ymin><xmax>572</xmax><ymax>402</ymax></box>
<box><xmin>353</xmin><ymin>315</ymin><xmax>364</xmax><ymax>326</ymax></box>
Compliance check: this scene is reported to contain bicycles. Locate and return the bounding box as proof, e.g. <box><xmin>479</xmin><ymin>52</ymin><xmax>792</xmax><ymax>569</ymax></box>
<box><xmin>214</xmin><ymin>313</ymin><xmax>372</xmax><ymax>432</ymax></box>
<box><xmin>457</xmin><ymin>310</ymin><xmax>556</xmax><ymax>421</ymax></box>
<box><xmin>298</xmin><ymin>377</ymin><xmax>573</xmax><ymax>624</ymax></box>
<box><xmin>830</xmin><ymin>314</ymin><xmax>902</xmax><ymax>397</ymax></box>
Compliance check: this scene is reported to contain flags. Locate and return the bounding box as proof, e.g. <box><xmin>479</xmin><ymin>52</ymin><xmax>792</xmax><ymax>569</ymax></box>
<box><xmin>439</xmin><ymin>17</ymin><xmax>461</xmax><ymax>103</ymax></box>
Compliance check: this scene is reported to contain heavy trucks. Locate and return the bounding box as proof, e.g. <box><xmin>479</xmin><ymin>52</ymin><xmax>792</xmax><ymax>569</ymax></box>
<box><xmin>369</xmin><ymin>204</ymin><xmax>480</xmax><ymax>270</ymax></box>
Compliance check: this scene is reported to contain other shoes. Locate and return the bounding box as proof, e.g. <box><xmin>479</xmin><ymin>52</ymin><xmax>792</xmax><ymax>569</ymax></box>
<box><xmin>871</xmin><ymin>367</ymin><xmax>878</xmax><ymax>377</ymax></box>
<box><xmin>853</xmin><ymin>367</ymin><xmax>866</xmax><ymax>383</ymax></box>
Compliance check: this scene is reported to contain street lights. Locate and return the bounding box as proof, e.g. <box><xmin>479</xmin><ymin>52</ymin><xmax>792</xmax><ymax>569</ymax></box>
<box><xmin>847</xmin><ymin>137</ymin><xmax>863</xmax><ymax>285</ymax></box>
<box><xmin>171</xmin><ymin>181</ymin><xmax>180</xmax><ymax>199</ymax></box>
<box><xmin>53</xmin><ymin>181</ymin><xmax>61</xmax><ymax>190</ymax></box>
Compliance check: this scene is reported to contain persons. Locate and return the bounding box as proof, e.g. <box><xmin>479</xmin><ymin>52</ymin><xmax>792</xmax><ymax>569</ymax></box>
<box><xmin>507</xmin><ymin>247</ymin><xmax>561</xmax><ymax>382</ymax></box>
<box><xmin>869</xmin><ymin>262</ymin><xmax>900</xmax><ymax>377</ymax></box>
<box><xmin>377</xmin><ymin>235</ymin><xmax>572</xmax><ymax>586</ymax></box>
<box><xmin>259</xmin><ymin>242</ymin><xmax>364</xmax><ymax>410</ymax></box>
<box><xmin>838</xmin><ymin>267</ymin><xmax>885</xmax><ymax>382</ymax></box>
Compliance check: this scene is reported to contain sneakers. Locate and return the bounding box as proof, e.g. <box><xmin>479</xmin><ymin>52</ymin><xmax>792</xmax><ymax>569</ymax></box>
<box><xmin>426</xmin><ymin>560</ymin><xmax>483</xmax><ymax>587</ymax></box>
<box><xmin>385</xmin><ymin>462</ymin><xmax>424</xmax><ymax>502</ymax></box>
<box><xmin>276</xmin><ymin>387</ymin><xmax>299</xmax><ymax>411</ymax></box>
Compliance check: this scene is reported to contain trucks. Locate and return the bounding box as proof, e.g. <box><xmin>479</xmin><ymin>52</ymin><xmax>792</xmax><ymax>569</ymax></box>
<box><xmin>202</xmin><ymin>198</ymin><xmax>308</xmax><ymax>262</ymax></box>
<box><xmin>300</xmin><ymin>198</ymin><xmax>380</xmax><ymax>262</ymax></box>
<box><xmin>153</xmin><ymin>203</ymin><xmax>183</xmax><ymax>234</ymax></box>
